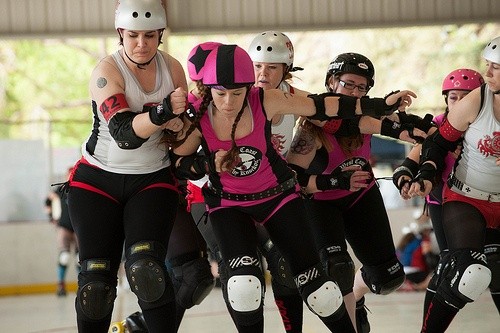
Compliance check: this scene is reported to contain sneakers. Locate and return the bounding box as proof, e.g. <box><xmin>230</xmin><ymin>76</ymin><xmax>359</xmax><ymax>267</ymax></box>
<box><xmin>353</xmin><ymin>296</ymin><xmax>371</xmax><ymax>333</ymax></box>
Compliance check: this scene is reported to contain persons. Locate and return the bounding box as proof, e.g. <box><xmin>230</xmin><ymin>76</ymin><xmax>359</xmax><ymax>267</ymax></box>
<box><xmin>67</xmin><ymin>0</ymin><xmax>500</xmax><ymax>333</ymax></box>
<box><xmin>46</xmin><ymin>167</ymin><xmax>80</xmax><ymax>296</ymax></box>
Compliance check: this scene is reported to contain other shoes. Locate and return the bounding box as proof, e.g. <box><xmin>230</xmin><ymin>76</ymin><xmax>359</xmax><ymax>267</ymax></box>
<box><xmin>58</xmin><ymin>288</ymin><xmax>65</xmax><ymax>296</ymax></box>
<box><xmin>214</xmin><ymin>278</ymin><xmax>221</xmax><ymax>287</ymax></box>
<box><xmin>106</xmin><ymin>320</ymin><xmax>130</xmax><ymax>333</ymax></box>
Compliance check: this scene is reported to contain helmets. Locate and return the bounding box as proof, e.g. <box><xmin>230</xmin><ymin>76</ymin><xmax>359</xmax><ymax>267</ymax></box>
<box><xmin>203</xmin><ymin>45</ymin><xmax>255</xmax><ymax>89</ymax></box>
<box><xmin>481</xmin><ymin>37</ymin><xmax>500</xmax><ymax>65</ymax></box>
<box><xmin>324</xmin><ymin>53</ymin><xmax>374</xmax><ymax>87</ymax></box>
<box><xmin>247</xmin><ymin>31</ymin><xmax>294</xmax><ymax>66</ymax></box>
<box><xmin>442</xmin><ymin>69</ymin><xmax>485</xmax><ymax>95</ymax></box>
<box><xmin>188</xmin><ymin>42</ymin><xmax>224</xmax><ymax>81</ymax></box>
<box><xmin>115</xmin><ymin>0</ymin><xmax>166</xmax><ymax>30</ymax></box>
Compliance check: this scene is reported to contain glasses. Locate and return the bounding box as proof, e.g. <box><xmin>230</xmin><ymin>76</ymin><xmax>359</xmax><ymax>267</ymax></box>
<box><xmin>338</xmin><ymin>80</ymin><xmax>369</xmax><ymax>91</ymax></box>
